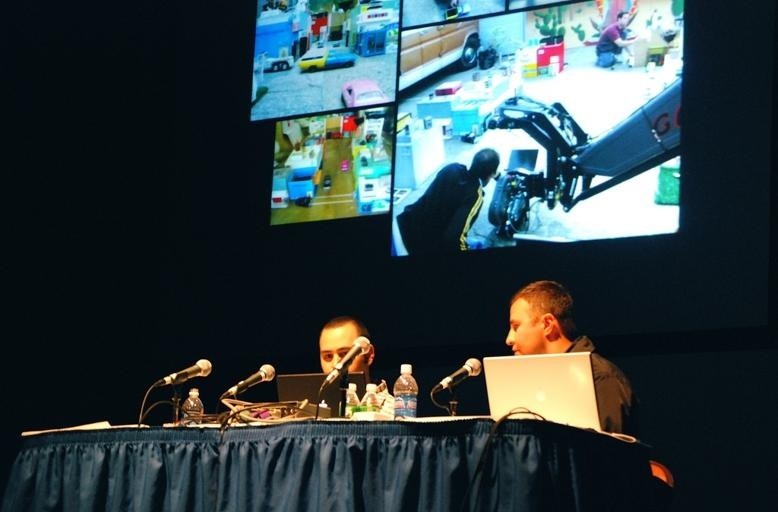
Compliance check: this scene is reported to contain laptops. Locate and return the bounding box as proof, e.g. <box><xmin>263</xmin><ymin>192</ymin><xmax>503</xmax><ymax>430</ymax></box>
<box><xmin>274</xmin><ymin>371</ymin><xmax>366</xmax><ymax>415</ymax></box>
<box><xmin>479</xmin><ymin>349</ymin><xmax>603</xmax><ymax>430</ymax></box>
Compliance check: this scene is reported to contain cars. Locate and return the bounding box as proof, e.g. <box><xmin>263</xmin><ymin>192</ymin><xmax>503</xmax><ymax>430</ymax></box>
<box><xmin>398</xmin><ymin>18</ymin><xmax>481</xmax><ymax>96</ymax></box>
<box><xmin>341</xmin><ymin>77</ymin><xmax>389</xmax><ymax>108</ymax></box>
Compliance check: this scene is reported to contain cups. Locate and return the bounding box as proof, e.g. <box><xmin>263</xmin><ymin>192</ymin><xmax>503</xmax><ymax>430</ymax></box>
<box><xmin>521</xmin><ymin>65</ymin><xmax>537</xmax><ymax>79</ymax></box>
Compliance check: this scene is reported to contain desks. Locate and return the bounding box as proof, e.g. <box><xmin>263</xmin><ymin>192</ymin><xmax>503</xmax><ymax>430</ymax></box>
<box><xmin>5</xmin><ymin>416</ymin><xmax>650</xmax><ymax>512</ymax></box>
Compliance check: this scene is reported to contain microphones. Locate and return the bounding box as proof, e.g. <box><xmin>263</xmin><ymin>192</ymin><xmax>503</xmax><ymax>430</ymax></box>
<box><xmin>227</xmin><ymin>362</ymin><xmax>277</xmax><ymax>398</ymax></box>
<box><xmin>168</xmin><ymin>358</ymin><xmax>214</xmax><ymax>386</ymax></box>
<box><xmin>435</xmin><ymin>355</ymin><xmax>483</xmax><ymax>394</ymax></box>
<box><xmin>321</xmin><ymin>333</ymin><xmax>370</xmax><ymax>388</ymax></box>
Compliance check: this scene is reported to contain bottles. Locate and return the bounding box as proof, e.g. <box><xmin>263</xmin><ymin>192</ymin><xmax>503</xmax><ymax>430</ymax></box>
<box><xmin>392</xmin><ymin>364</ymin><xmax>416</xmax><ymax>419</ymax></box>
<box><xmin>548</xmin><ymin>56</ymin><xmax>560</xmax><ymax>76</ymax></box>
<box><xmin>337</xmin><ymin>382</ymin><xmax>360</xmax><ymax>415</ymax></box>
<box><xmin>536</xmin><ymin>36</ymin><xmax>564</xmax><ymax>75</ymax></box>
<box><xmin>310</xmin><ymin>10</ymin><xmax>344</xmax><ymax>45</ymax></box>
<box><xmin>182</xmin><ymin>387</ymin><xmax>204</xmax><ymax>425</ymax></box>
<box><xmin>358</xmin><ymin>383</ymin><xmax>380</xmax><ymax>413</ymax></box>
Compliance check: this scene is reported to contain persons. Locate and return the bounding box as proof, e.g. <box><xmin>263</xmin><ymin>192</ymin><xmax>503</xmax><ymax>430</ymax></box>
<box><xmin>506</xmin><ymin>280</ymin><xmax>651</xmax><ymax>442</ymax></box>
<box><xmin>396</xmin><ymin>147</ymin><xmax>500</xmax><ymax>257</ymax></box>
<box><xmin>319</xmin><ymin>316</ymin><xmax>395</xmax><ymax>421</ymax></box>
<box><xmin>594</xmin><ymin>12</ymin><xmax>636</xmax><ymax>68</ymax></box>
